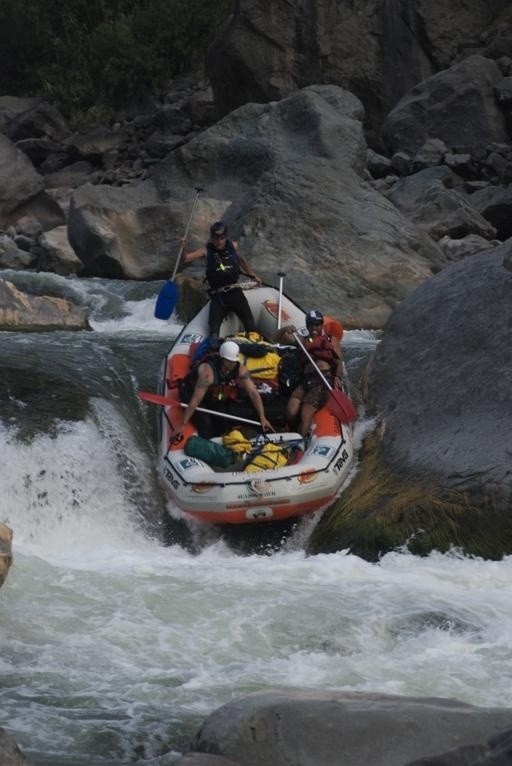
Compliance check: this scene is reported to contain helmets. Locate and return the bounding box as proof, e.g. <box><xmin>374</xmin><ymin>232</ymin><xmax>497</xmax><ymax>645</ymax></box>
<box><xmin>220</xmin><ymin>342</ymin><xmax>239</xmax><ymax>361</ymax></box>
<box><xmin>306</xmin><ymin>311</ymin><xmax>323</xmax><ymax>324</ymax></box>
<box><xmin>211</xmin><ymin>222</ymin><xmax>227</xmax><ymax>240</ymax></box>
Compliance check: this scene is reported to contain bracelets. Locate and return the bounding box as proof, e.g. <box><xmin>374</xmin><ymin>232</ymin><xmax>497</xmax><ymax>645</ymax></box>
<box><xmin>334</xmin><ymin>374</ymin><xmax>343</xmax><ymax>380</ymax></box>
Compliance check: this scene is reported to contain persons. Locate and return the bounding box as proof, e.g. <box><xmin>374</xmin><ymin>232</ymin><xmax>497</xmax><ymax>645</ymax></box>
<box><xmin>180</xmin><ymin>221</ymin><xmax>262</xmax><ymax>341</ymax></box>
<box><xmin>272</xmin><ymin>310</ymin><xmax>344</xmax><ymax>438</ymax></box>
<box><xmin>169</xmin><ymin>342</ymin><xmax>278</xmax><ymax>446</ymax></box>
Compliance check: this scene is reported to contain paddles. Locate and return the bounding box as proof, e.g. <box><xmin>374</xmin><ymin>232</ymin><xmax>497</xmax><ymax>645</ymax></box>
<box><xmin>155</xmin><ymin>187</ymin><xmax>207</xmax><ymax>319</ymax></box>
<box><xmin>139</xmin><ymin>393</ymin><xmax>262</xmax><ymax>426</ymax></box>
<box><xmin>292</xmin><ymin>332</ymin><xmax>358</xmax><ymax>423</ymax></box>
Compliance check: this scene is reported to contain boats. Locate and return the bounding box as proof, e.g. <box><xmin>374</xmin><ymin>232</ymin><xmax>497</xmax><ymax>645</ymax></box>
<box><xmin>156</xmin><ymin>278</ymin><xmax>354</xmax><ymax>526</ymax></box>
